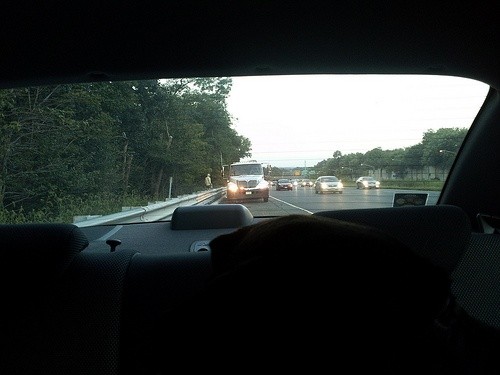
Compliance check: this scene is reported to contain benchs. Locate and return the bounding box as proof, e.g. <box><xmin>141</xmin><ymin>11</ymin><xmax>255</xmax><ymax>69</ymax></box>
<box><xmin>0</xmin><ymin>205</ymin><xmax>500</xmax><ymax>375</ymax></box>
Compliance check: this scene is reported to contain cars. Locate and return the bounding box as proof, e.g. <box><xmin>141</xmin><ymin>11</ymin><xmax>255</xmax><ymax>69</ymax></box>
<box><xmin>269</xmin><ymin>177</ymin><xmax>312</xmax><ymax>184</ymax></box>
<box><xmin>356</xmin><ymin>176</ymin><xmax>380</xmax><ymax>190</ymax></box>
<box><xmin>314</xmin><ymin>175</ymin><xmax>344</xmax><ymax>195</ymax></box>
<box><xmin>300</xmin><ymin>178</ymin><xmax>313</xmax><ymax>187</ymax></box>
<box><xmin>277</xmin><ymin>178</ymin><xmax>293</xmax><ymax>191</ymax></box>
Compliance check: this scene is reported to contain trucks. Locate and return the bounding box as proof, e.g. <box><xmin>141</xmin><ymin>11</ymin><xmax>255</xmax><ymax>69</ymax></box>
<box><xmin>220</xmin><ymin>161</ymin><xmax>273</xmax><ymax>204</ymax></box>
